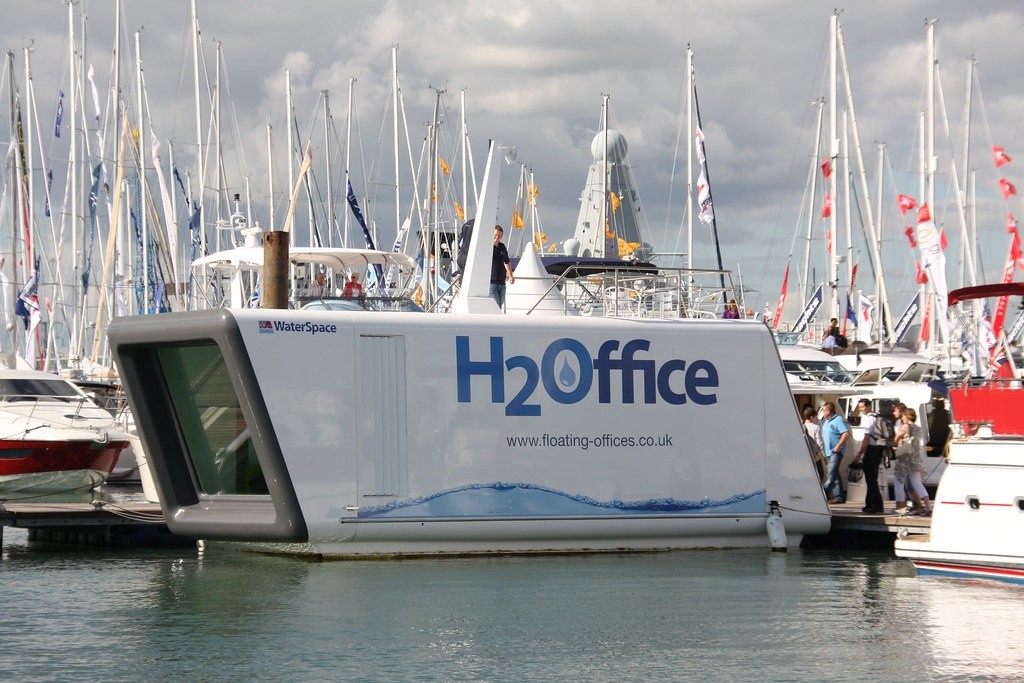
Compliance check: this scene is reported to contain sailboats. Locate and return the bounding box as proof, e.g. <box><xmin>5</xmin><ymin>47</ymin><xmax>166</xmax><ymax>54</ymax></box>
<box><xmin>0</xmin><ymin>0</ymin><xmax>1024</xmax><ymax>501</ymax></box>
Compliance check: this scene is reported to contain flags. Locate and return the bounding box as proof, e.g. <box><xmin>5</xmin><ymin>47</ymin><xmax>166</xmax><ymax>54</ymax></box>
<box><xmin>819</xmin><ymin>145</ymin><xmax>1024</xmax><ymax>294</ymax></box>
<box><xmin>14</xmin><ymin>256</ymin><xmax>42</xmax><ymax>335</ymax></box>
<box><xmin>695</xmin><ymin>125</ymin><xmax>715</xmax><ymax>224</ymax></box>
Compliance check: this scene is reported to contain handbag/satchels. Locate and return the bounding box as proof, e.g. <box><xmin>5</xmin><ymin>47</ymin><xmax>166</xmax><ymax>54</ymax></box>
<box><xmin>895</xmin><ymin>424</ymin><xmax>914</xmax><ymax>459</ymax></box>
<box><xmin>847</xmin><ymin>462</ymin><xmax>863</xmax><ymax>482</ymax></box>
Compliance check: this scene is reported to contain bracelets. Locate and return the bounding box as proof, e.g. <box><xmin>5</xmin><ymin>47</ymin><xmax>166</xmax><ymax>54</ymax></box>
<box><xmin>857</xmin><ymin>453</ymin><xmax>862</xmax><ymax>457</ymax></box>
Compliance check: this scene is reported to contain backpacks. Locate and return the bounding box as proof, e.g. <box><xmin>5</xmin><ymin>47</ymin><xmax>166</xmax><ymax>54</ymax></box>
<box><xmin>867</xmin><ymin>413</ymin><xmax>896</xmax><ymax>469</ymax></box>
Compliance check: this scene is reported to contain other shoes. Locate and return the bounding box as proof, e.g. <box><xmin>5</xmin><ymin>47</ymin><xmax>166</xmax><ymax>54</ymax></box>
<box><xmin>828</xmin><ymin>496</ymin><xmax>932</xmax><ymax>516</ymax></box>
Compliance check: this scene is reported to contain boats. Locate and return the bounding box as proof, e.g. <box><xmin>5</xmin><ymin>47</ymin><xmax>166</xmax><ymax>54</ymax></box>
<box><xmin>894</xmin><ymin>279</ymin><xmax>1023</xmax><ymax>589</ymax></box>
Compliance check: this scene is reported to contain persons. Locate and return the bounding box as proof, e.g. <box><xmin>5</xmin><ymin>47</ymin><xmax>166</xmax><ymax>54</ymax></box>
<box><xmin>456</xmin><ymin>218</ymin><xmax>475</xmax><ymax>287</ymax></box>
<box><xmin>822</xmin><ymin>318</ymin><xmax>847</xmax><ymax>350</ymax></box>
<box><xmin>340</xmin><ymin>272</ymin><xmax>363</xmax><ymax>305</ymax></box>
<box><xmin>722</xmin><ymin>299</ymin><xmax>740</xmax><ymax>319</ymax></box>
<box><xmin>489</xmin><ymin>225</ymin><xmax>515</xmax><ymax>309</ymax></box>
<box><xmin>890</xmin><ymin>403</ymin><xmax>933</xmax><ymax>516</ymax></box>
<box><xmin>308</xmin><ymin>272</ymin><xmax>325</xmax><ymax>298</ymax></box>
<box><xmin>802</xmin><ymin>398</ymin><xmax>849</xmax><ymax>505</ymax></box>
<box><xmin>853</xmin><ymin>399</ymin><xmax>888</xmax><ymax>513</ymax></box>
